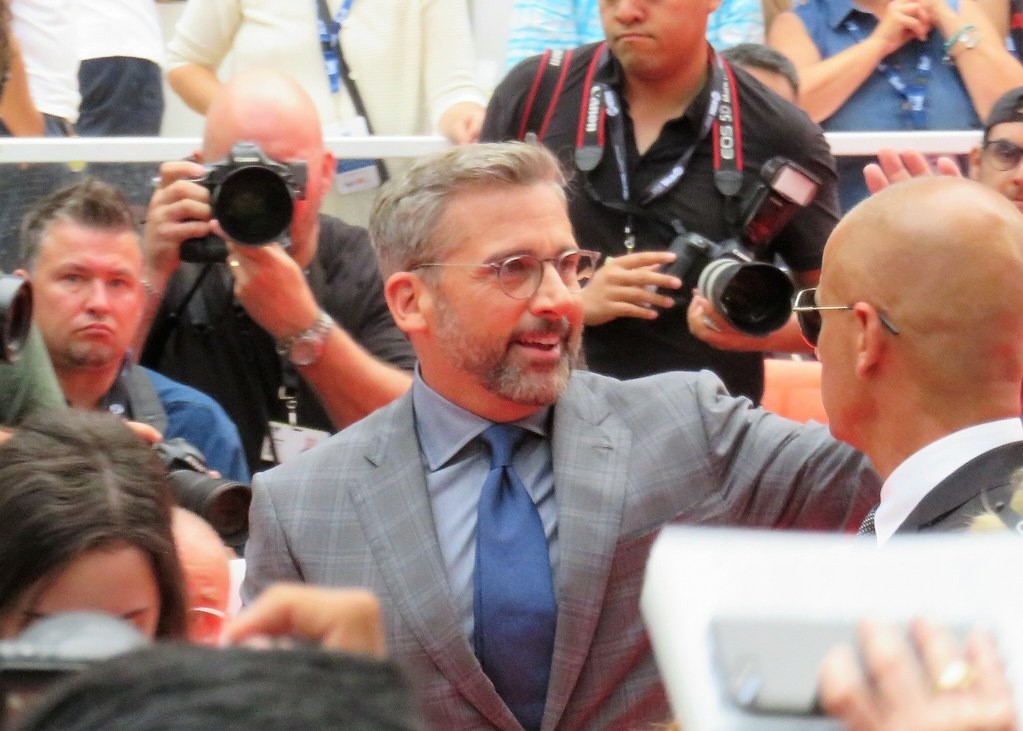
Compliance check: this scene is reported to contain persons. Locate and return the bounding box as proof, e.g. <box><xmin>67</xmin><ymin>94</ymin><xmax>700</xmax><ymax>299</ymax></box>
<box><xmin>0</xmin><ymin>0</ymin><xmax>1023</xmax><ymax>731</ymax></box>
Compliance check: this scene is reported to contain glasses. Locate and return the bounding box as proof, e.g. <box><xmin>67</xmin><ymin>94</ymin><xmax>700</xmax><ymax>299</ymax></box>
<box><xmin>986</xmin><ymin>140</ymin><xmax>1023</xmax><ymax>171</ymax></box>
<box><xmin>408</xmin><ymin>249</ymin><xmax>600</xmax><ymax>299</ymax></box>
<box><xmin>792</xmin><ymin>288</ymin><xmax>900</xmax><ymax>348</ymax></box>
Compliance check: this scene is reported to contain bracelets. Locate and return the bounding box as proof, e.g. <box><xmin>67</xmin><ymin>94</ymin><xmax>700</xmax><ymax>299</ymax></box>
<box><xmin>942</xmin><ymin>24</ymin><xmax>977</xmax><ymax>63</ymax></box>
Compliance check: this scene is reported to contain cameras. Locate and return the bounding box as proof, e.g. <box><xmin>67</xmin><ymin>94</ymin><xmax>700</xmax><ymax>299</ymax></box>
<box><xmin>642</xmin><ymin>155</ymin><xmax>823</xmax><ymax>338</ymax></box>
<box><xmin>145</xmin><ymin>436</ymin><xmax>254</xmax><ymax>547</ymax></box>
<box><xmin>175</xmin><ymin>140</ymin><xmax>306</xmax><ymax>265</ymax></box>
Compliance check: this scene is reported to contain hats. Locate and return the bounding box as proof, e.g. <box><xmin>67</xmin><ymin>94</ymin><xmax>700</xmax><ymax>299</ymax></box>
<box><xmin>986</xmin><ymin>85</ymin><xmax>1023</xmax><ymax>130</ymax></box>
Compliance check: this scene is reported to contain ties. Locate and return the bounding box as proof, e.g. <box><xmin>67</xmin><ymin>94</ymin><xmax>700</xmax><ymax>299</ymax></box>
<box><xmin>852</xmin><ymin>504</ymin><xmax>879</xmax><ymax>543</ymax></box>
<box><xmin>474</xmin><ymin>424</ymin><xmax>558</xmax><ymax>731</ymax></box>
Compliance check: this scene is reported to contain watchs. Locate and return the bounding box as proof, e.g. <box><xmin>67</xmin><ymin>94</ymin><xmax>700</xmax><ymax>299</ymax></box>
<box><xmin>274</xmin><ymin>310</ymin><xmax>332</xmax><ymax>366</ymax></box>
